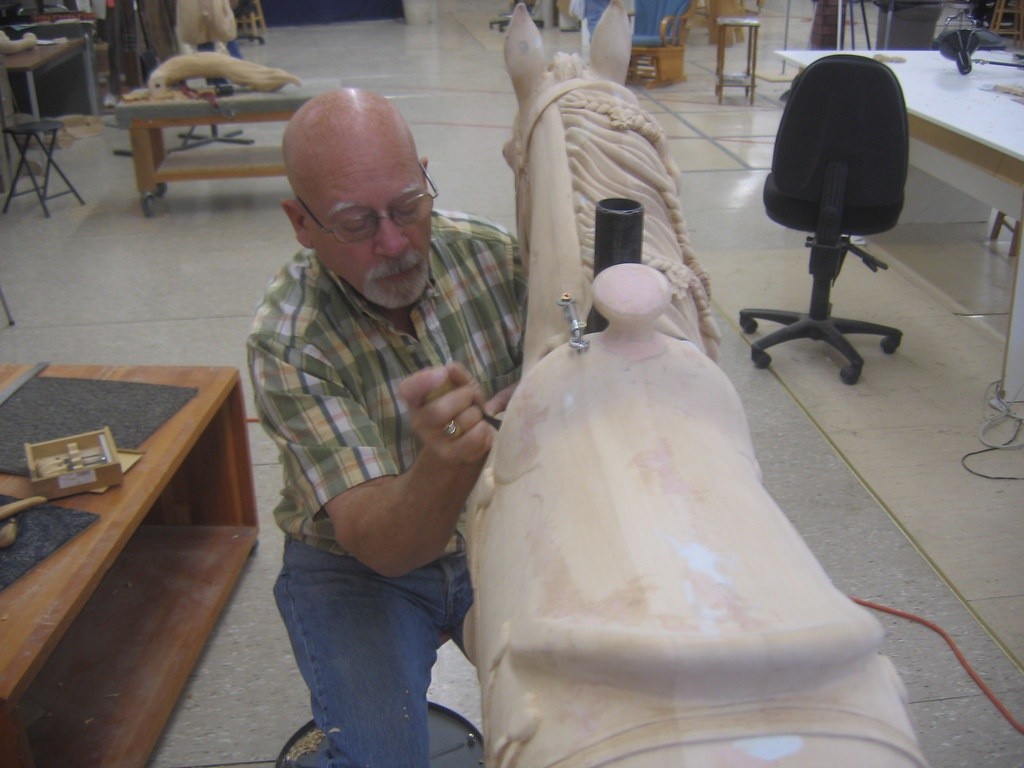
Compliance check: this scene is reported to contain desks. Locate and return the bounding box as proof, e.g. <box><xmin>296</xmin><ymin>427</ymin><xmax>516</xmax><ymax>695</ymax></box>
<box><xmin>773</xmin><ymin>48</ymin><xmax>1023</xmax><ymax>404</ymax></box>
<box><xmin>116</xmin><ymin>78</ymin><xmax>341</xmax><ymax>217</ymax></box>
<box><xmin>0</xmin><ymin>37</ymin><xmax>88</xmax><ymax>151</ymax></box>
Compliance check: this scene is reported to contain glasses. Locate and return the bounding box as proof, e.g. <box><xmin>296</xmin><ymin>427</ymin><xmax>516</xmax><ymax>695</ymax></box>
<box><xmin>295</xmin><ymin>162</ymin><xmax>438</xmax><ymax>243</ymax></box>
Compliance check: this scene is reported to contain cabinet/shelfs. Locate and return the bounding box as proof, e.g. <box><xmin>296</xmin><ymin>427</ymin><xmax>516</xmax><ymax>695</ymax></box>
<box><xmin>0</xmin><ymin>362</ymin><xmax>262</xmax><ymax>768</ymax></box>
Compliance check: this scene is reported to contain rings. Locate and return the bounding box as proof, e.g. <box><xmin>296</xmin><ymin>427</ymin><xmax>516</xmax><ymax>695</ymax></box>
<box><xmin>443</xmin><ymin>421</ymin><xmax>458</xmax><ymax>439</ymax></box>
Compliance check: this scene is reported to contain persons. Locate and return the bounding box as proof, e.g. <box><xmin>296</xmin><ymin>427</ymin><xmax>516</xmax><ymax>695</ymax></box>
<box><xmin>176</xmin><ymin>0</ymin><xmax>243</xmax><ymax>85</ymax></box>
<box><xmin>246</xmin><ymin>88</ymin><xmax>531</xmax><ymax>768</ymax></box>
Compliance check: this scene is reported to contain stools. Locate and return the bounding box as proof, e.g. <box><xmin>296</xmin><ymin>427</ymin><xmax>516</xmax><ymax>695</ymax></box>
<box><xmin>714</xmin><ymin>15</ymin><xmax>759</xmax><ymax>104</ymax></box>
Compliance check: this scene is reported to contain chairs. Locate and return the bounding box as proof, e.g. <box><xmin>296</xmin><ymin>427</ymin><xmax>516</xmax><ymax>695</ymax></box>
<box><xmin>0</xmin><ymin>90</ymin><xmax>86</xmax><ymax>217</ymax></box>
<box><xmin>620</xmin><ymin>1</ymin><xmax>700</xmax><ymax>90</ymax></box>
<box><xmin>739</xmin><ymin>53</ymin><xmax>909</xmax><ymax>386</ymax></box>
